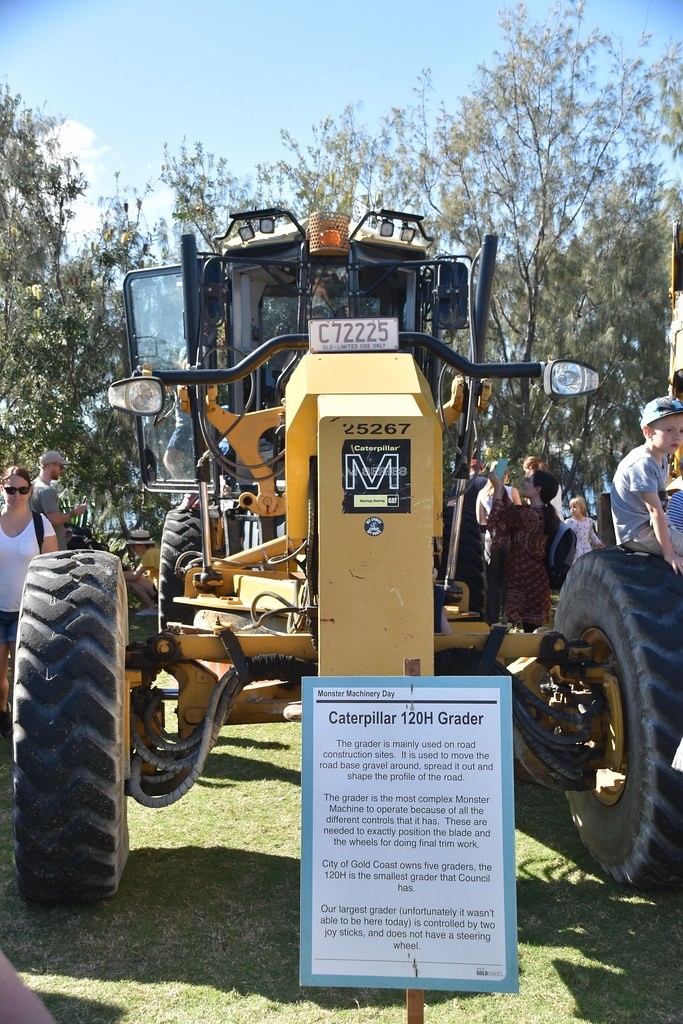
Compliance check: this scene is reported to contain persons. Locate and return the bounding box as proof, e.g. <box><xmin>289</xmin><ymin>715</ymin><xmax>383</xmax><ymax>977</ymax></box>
<box><xmin>161</xmin><ymin>346</ymin><xmax>212</xmax><ymax>514</ymax></box>
<box><xmin>0</xmin><ymin>451</ymin><xmax>90</xmax><ymax>740</ymax></box>
<box><xmin>431</xmin><ymin>395</ymin><xmax>683</xmax><ymax>634</ymax></box>
<box><xmin>123</xmin><ymin>529</ymin><xmax>161</xmax><ymax>616</ymax></box>
<box><xmin>309</xmin><ymin>278</ymin><xmax>352</xmax><ymax>321</ymax></box>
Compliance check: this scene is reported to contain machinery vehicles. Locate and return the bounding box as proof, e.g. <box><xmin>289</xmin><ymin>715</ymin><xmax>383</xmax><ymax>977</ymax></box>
<box><xmin>12</xmin><ymin>206</ymin><xmax>683</xmax><ymax>910</ymax></box>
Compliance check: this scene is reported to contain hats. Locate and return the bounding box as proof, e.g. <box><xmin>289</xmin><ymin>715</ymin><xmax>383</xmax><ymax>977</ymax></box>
<box><xmin>40</xmin><ymin>451</ymin><xmax>71</xmax><ymax>465</ymax></box>
<box><xmin>471</xmin><ymin>459</ymin><xmax>483</xmax><ymax>469</ymax></box>
<box><xmin>640</xmin><ymin>396</ymin><xmax>682</xmax><ymax>430</ymax></box>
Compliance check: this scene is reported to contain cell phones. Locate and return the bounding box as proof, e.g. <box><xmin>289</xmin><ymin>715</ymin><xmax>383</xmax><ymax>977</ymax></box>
<box><xmin>496</xmin><ymin>458</ymin><xmax>508</xmax><ymax>479</ymax></box>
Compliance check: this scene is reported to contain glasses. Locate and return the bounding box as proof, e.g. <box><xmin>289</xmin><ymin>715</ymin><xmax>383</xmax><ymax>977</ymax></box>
<box><xmin>4</xmin><ymin>485</ymin><xmax>29</xmax><ymax>494</ymax></box>
<box><xmin>53</xmin><ymin>463</ymin><xmax>64</xmax><ymax>469</ymax></box>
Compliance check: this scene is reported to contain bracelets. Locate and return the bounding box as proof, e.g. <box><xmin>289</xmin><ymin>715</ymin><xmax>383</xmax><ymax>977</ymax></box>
<box><xmin>69</xmin><ymin>511</ymin><xmax>75</xmax><ymax>517</ymax></box>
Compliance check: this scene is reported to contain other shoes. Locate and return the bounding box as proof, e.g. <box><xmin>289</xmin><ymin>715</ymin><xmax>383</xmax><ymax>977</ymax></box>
<box><xmin>0</xmin><ymin>701</ymin><xmax>12</xmax><ymax>740</ymax></box>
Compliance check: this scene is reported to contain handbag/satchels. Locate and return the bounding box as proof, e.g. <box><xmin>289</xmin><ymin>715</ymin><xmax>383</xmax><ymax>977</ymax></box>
<box><xmin>543</xmin><ymin>519</ymin><xmax>578</xmax><ymax>588</ymax></box>
